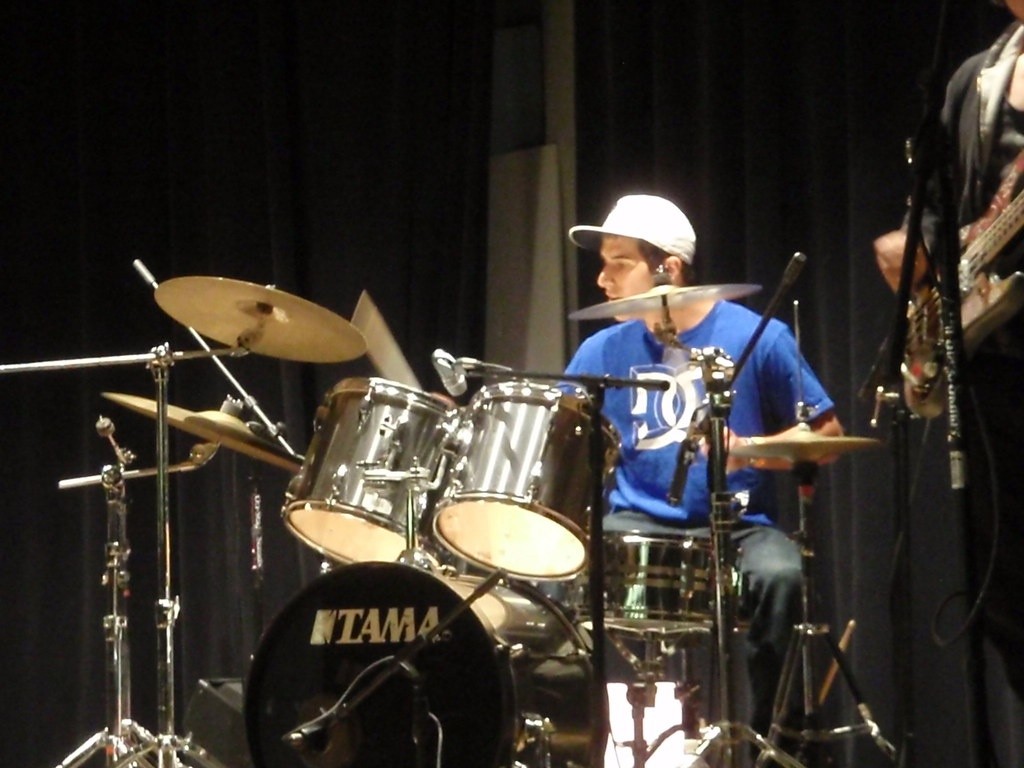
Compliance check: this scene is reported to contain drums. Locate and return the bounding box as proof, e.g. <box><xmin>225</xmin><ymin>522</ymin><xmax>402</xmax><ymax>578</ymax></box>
<box><xmin>601</xmin><ymin>528</ymin><xmax>744</xmax><ymax>636</ymax></box>
<box><xmin>430</xmin><ymin>379</ymin><xmax>623</xmax><ymax>582</ymax></box>
<box><xmin>240</xmin><ymin>556</ymin><xmax>605</xmax><ymax>768</ymax></box>
<box><xmin>279</xmin><ymin>376</ymin><xmax>456</xmax><ymax>566</ymax></box>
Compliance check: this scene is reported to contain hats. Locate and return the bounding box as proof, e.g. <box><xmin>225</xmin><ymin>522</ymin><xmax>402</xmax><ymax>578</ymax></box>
<box><xmin>568</xmin><ymin>194</ymin><xmax>696</xmax><ymax>265</ymax></box>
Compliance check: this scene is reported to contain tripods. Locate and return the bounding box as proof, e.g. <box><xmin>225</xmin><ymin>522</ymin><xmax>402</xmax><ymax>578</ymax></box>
<box><xmin>653</xmin><ymin>295</ymin><xmax>897</xmax><ymax>768</ymax></box>
<box><xmin>0</xmin><ymin>322</ymin><xmax>264</xmax><ymax>768</ymax></box>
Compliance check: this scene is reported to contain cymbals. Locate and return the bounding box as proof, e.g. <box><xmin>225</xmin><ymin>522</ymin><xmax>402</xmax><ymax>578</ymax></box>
<box><xmin>567</xmin><ymin>282</ymin><xmax>764</xmax><ymax>322</ymax></box>
<box><xmin>99</xmin><ymin>389</ymin><xmax>304</xmax><ymax>472</ymax></box>
<box><xmin>153</xmin><ymin>273</ymin><xmax>370</xmax><ymax>365</ymax></box>
<box><xmin>726</xmin><ymin>422</ymin><xmax>885</xmax><ymax>463</ymax></box>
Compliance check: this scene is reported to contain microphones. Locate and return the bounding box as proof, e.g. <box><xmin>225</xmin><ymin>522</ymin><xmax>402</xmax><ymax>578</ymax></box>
<box><xmin>283</xmin><ymin>724</ymin><xmax>325</xmax><ymax>749</ymax></box>
<box><xmin>431</xmin><ymin>349</ymin><xmax>468</xmax><ymax>396</ymax></box>
<box><xmin>667</xmin><ymin>410</ymin><xmax>698</xmax><ymax>507</ymax></box>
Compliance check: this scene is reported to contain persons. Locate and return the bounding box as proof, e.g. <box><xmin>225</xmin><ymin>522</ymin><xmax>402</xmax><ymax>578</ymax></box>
<box><xmin>871</xmin><ymin>1</ymin><xmax>1024</xmax><ymax>428</ymax></box>
<box><xmin>551</xmin><ymin>194</ymin><xmax>844</xmax><ymax>768</ymax></box>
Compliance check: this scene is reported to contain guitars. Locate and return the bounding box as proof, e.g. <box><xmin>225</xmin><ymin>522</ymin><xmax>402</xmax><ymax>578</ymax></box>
<box><xmin>901</xmin><ymin>187</ymin><xmax>1024</xmax><ymax>418</ymax></box>
